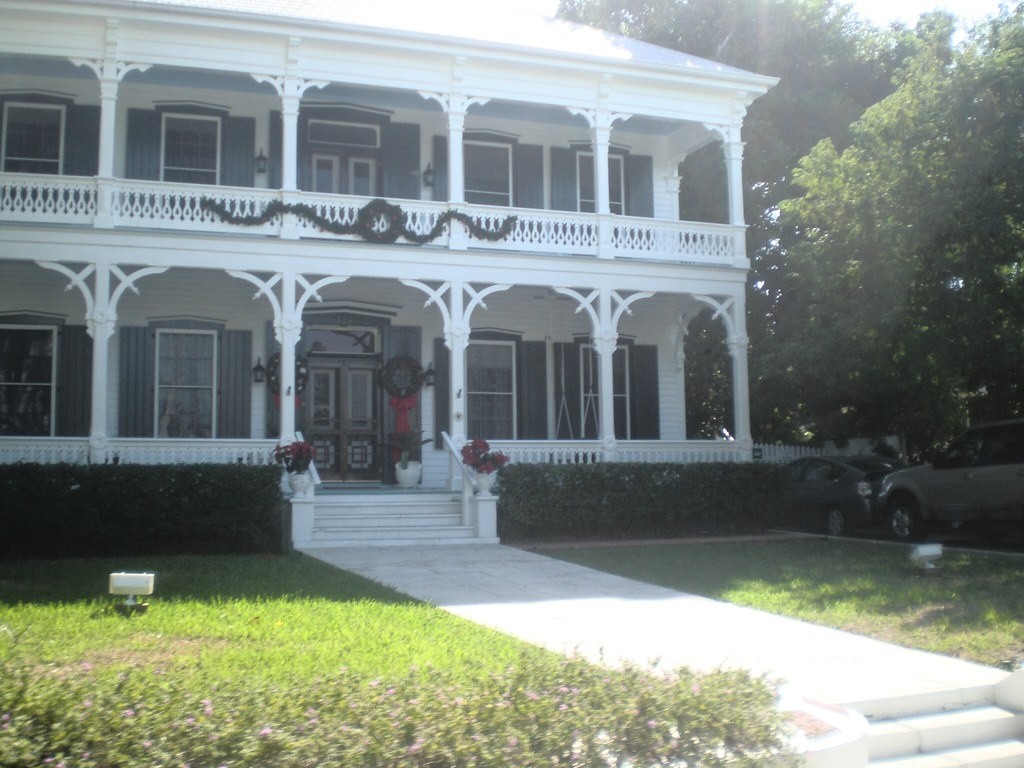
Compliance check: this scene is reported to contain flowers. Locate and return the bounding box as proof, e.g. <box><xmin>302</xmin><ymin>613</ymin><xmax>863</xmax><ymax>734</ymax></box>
<box><xmin>461</xmin><ymin>439</ymin><xmax>512</xmax><ymax>474</ymax></box>
<box><xmin>275</xmin><ymin>442</ymin><xmax>317</xmax><ymax>473</ymax></box>
<box><xmin>381</xmin><ymin>355</ymin><xmax>423</xmax><ymax>400</ymax></box>
<box><xmin>265</xmin><ymin>353</ymin><xmax>308</xmax><ymax>396</ymax></box>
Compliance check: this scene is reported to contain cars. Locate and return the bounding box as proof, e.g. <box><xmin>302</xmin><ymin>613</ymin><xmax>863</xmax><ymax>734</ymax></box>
<box><xmin>759</xmin><ymin>453</ymin><xmax>911</xmax><ymax>537</ymax></box>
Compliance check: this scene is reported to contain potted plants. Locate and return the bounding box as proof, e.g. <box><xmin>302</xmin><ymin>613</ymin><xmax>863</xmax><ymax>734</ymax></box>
<box><xmin>388</xmin><ymin>431</ymin><xmax>434</xmax><ymax>485</ymax></box>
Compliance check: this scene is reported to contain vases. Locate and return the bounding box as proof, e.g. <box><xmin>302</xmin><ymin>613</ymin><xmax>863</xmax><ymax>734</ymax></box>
<box><xmin>474</xmin><ymin>469</ymin><xmax>499</xmax><ymax>497</ymax></box>
<box><xmin>285</xmin><ymin>471</ymin><xmax>310</xmax><ymax>499</ymax></box>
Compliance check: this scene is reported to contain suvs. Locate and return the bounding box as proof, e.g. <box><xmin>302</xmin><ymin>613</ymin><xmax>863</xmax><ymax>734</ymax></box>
<box><xmin>875</xmin><ymin>417</ymin><xmax>1024</xmax><ymax>548</ymax></box>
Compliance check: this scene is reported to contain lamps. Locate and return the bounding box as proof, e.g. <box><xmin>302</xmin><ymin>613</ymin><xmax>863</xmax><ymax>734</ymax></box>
<box><xmin>252</xmin><ymin>357</ymin><xmax>266</xmax><ymax>382</ymax></box>
<box><xmin>911</xmin><ymin>544</ymin><xmax>943</xmax><ymax>570</ymax></box>
<box><xmin>109</xmin><ymin>573</ymin><xmax>155</xmax><ymax>605</ymax></box>
<box><xmin>423</xmin><ymin>162</ymin><xmax>434</xmax><ymax>186</ymax></box>
<box><xmin>423</xmin><ymin>360</ymin><xmax>436</xmax><ymax>385</ymax></box>
<box><xmin>253</xmin><ymin>148</ymin><xmax>269</xmax><ymax>172</ymax></box>
